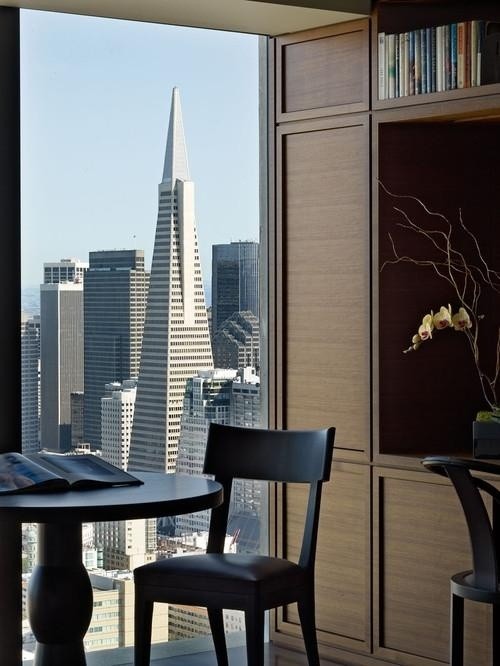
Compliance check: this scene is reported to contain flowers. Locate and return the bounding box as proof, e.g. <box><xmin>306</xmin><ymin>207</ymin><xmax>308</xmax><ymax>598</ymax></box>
<box><xmin>370</xmin><ymin>174</ymin><xmax>500</xmax><ymax>420</ymax></box>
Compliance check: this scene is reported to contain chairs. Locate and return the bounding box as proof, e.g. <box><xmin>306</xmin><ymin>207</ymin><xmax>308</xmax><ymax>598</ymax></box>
<box><xmin>421</xmin><ymin>457</ymin><xmax>500</xmax><ymax>665</ymax></box>
<box><xmin>134</xmin><ymin>423</ymin><xmax>336</xmax><ymax>665</ymax></box>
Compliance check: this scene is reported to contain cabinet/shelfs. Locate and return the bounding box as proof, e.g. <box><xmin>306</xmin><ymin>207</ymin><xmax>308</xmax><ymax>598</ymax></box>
<box><xmin>369</xmin><ymin>0</ymin><xmax>500</xmax><ymax>109</ymax></box>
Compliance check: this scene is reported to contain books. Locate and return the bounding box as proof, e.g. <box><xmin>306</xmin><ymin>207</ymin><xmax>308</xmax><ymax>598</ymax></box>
<box><xmin>378</xmin><ymin>18</ymin><xmax>500</xmax><ymax>101</ymax></box>
<box><xmin>0</xmin><ymin>449</ymin><xmax>145</xmax><ymax>493</ymax></box>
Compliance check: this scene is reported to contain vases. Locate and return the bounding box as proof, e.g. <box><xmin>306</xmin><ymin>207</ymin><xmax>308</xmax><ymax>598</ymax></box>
<box><xmin>472</xmin><ymin>420</ymin><xmax>500</xmax><ymax>461</ymax></box>
